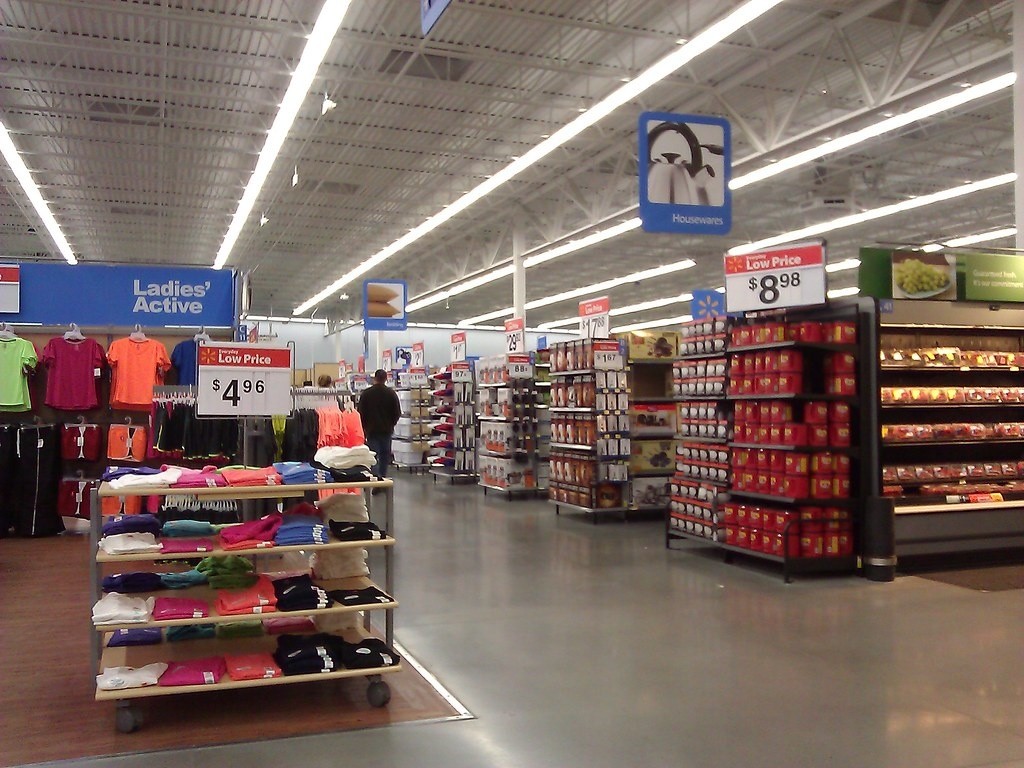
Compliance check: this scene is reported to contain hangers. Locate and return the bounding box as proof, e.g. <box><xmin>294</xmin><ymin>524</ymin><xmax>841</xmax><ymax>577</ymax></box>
<box><xmin>110</xmin><ymin>417</ymin><xmax>145</xmax><ymax>431</ymax></box>
<box><xmin>63</xmin><ymin>469</ymin><xmax>94</xmax><ymax>484</ymax></box>
<box><xmin>65</xmin><ymin>416</ymin><xmax>98</xmax><ymax>429</ymax></box>
<box><xmin>0</xmin><ymin>322</ymin><xmax>18</xmax><ymax>339</ymax></box>
<box><xmin>130</xmin><ymin>324</ymin><xmax>148</xmax><ymax>341</ymax></box>
<box><xmin>65</xmin><ymin>324</ymin><xmax>85</xmax><ymax>342</ymax></box>
<box><xmin>161</xmin><ymin>493</ymin><xmax>238</xmax><ymax>513</ymax></box>
<box><xmin>20</xmin><ymin>416</ymin><xmax>53</xmax><ymax>428</ymax></box>
<box><xmin>151</xmin><ymin>384</ymin><xmax>197</xmax><ymax>407</ymax></box>
<box><xmin>291</xmin><ymin>385</ymin><xmax>358</xmax><ymax>415</ymax></box>
<box><xmin>193</xmin><ymin>326</ymin><xmax>212</xmax><ymax>343</ymax></box>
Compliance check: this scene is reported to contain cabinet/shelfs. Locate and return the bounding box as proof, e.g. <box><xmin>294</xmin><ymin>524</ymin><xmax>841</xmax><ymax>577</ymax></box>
<box><xmin>628</xmin><ymin>357</ymin><xmax>678</xmax><ymax>522</ymax></box>
<box><xmin>89</xmin><ymin>479</ymin><xmax>399</xmax><ymax>729</ymax></box>
<box><xmin>477</xmin><ymin>354</ymin><xmax>537</xmax><ymax>502</ymax></box>
<box><xmin>392</xmin><ymin>369</ymin><xmax>435</xmax><ymax>477</ymax></box>
<box><xmin>428</xmin><ymin>360</ymin><xmax>476</xmax><ymax>484</ymax></box>
<box><xmin>533</xmin><ymin>362</ymin><xmax>551</xmax><ymax>496</ymax></box>
<box><xmin>667</xmin><ymin>308</ymin><xmax>860</xmax><ymax>584</ymax></box>
<box><xmin>548</xmin><ymin>338</ymin><xmax>629</xmax><ymax>520</ymax></box>
<box><xmin>860</xmin><ymin>297</ymin><xmax>1024</xmax><ymax>582</ymax></box>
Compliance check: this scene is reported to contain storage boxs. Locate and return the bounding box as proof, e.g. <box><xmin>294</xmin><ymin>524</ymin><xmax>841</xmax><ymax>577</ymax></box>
<box><xmin>629</xmin><ymin>316</ymin><xmax>735</xmax><ymax>543</ymax></box>
<box><xmin>536</xmin><ymin>339</ymin><xmax>631</xmax><ymax>509</ymax></box>
<box><xmin>478</xmin><ymin>388</ymin><xmax>533</xmax><ymax>489</ymax></box>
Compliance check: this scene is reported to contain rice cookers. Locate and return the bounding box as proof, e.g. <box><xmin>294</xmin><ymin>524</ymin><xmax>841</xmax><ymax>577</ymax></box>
<box><xmin>505</xmin><ymin>469</ymin><xmax>522</xmax><ymax>484</ymax></box>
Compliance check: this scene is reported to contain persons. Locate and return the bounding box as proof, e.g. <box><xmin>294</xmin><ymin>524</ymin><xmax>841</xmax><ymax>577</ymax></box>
<box><xmin>357</xmin><ymin>369</ymin><xmax>401</xmax><ymax>495</ymax></box>
<box><xmin>317</xmin><ymin>375</ymin><xmax>342</xmax><ymax>411</ymax></box>
